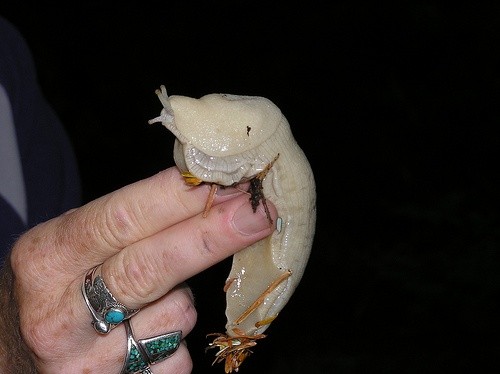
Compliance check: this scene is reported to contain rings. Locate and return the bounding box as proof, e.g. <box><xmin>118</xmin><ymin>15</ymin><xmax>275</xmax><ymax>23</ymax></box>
<box><xmin>81</xmin><ymin>263</ymin><xmax>140</xmax><ymax>335</ymax></box>
<box><xmin>120</xmin><ymin>320</ymin><xmax>182</xmax><ymax>374</ymax></box>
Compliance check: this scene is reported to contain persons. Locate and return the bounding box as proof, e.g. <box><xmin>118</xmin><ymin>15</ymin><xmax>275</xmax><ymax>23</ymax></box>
<box><xmin>0</xmin><ymin>14</ymin><xmax>277</xmax><ymax>373</ymax></box>
